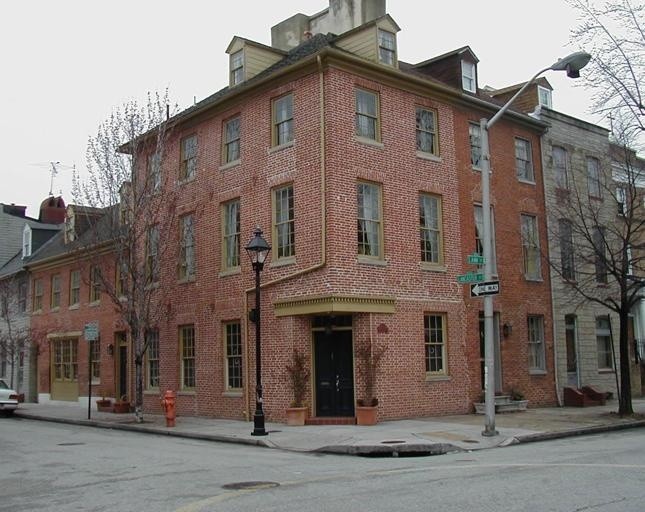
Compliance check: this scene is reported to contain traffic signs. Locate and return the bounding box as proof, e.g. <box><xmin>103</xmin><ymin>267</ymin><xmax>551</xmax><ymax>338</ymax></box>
<box><xmin>470</xmin><ymin>281</ymin><xmax>500</xmax><ymax>297</ymax></box>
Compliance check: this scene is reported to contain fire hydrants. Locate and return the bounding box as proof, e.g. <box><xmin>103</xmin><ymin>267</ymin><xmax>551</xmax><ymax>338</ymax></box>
<box><xmin>160</xmin><ymin>390</ymin><xmax>177</xmax><ymax>427</ymax></box>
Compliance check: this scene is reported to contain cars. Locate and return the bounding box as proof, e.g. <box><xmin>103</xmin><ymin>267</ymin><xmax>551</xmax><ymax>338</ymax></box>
<box><xmin>0</xmin><ymin>378</ymin><xmax>19</xmax><ymax>416</ymax></box>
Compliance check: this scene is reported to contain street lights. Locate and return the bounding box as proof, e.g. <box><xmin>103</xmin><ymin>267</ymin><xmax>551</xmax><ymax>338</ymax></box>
<box><xmin>481</xmin><ymin>51</ymin><xmax>591</xmax><ymax>437</ymax></box>
<box><xmin>245</xmin><ymin>225</ymin><xmax>272</xmax><ymax>436</ymax></box>
